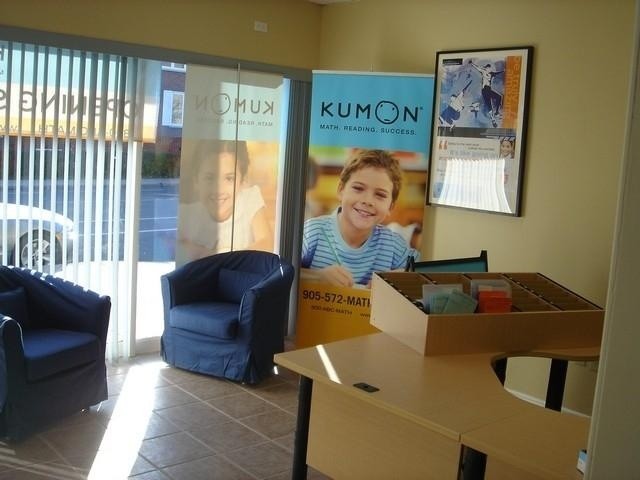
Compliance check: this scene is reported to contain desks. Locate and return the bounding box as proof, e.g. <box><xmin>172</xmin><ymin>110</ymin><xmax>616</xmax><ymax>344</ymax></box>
<box><xmin>273</xmin><ymin>331</ymin><xmax>602</xmax><ymax>480</ymax></box>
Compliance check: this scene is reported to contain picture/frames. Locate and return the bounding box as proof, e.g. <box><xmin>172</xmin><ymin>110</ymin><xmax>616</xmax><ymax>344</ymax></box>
<box><xmin>425</xmin><ymin>45</ymin><xmax>533</xmax><ymax>216</ymax></box>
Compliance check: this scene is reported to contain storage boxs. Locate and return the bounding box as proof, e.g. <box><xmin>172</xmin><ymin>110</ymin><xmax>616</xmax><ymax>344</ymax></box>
<box><xmin>368</xmin><ymin>270</ymin><xmax>603</xmax><ymax>356</ymax></box>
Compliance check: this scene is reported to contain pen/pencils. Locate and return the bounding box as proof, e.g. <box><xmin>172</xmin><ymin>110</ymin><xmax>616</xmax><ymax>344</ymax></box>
<box><xmin>320</xmin><ymin>226</ymin><xmax>342</xmax><ymax>265</ymax></box>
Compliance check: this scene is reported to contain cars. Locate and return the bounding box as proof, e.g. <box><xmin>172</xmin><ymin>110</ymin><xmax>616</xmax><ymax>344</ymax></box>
<box><xmin>0</xmin><ymin>200</ymin><xmax>75</xmax><ymax>270</ymax></box>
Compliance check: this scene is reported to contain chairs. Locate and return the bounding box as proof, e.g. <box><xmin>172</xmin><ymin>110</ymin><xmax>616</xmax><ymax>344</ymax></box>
<box><xmin>409</xmin><ymin>250</ymin><xmax>488</xmax><ymax>272</ymax></box>
<box><xmin>0</xmin><ymin>265</ymin><xmax>111</xmax><ymax>444</ymax></box>
<box><xmin>160</xmin><ymin>249</ymin><xmax>294</xmax><ymax>387</ymax></box>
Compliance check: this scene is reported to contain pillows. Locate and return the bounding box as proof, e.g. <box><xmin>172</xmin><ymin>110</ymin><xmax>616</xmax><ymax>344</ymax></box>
<box><xmin>216</xmin><ymin>267</ymin><xmax>264</xmax><ymax>304</ymax></box>
<box><xmin>0</xmin><ymin>286</ymin><xmax>32</xmax><ymax>327</ymax></box>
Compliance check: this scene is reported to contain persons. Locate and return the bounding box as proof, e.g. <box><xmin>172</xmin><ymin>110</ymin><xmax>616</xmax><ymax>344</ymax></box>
<box><xmin>468</xmin><ymin>100</ymin><xmax>480</xmax><ymax>117</ymax></box>
<box><xmin>176</xmin><ymin>138</ymin><xmax>274</xmax><ymax>263</ymax></box>
<box><xmin>501</xmin><ymin>135</ymin><xmax>515</xmax><ymax>160</ymax></box>
<box><xmin>438</xmin><ymin>80</ymin><xmax>473</xmax><ymax>132</ymax></box>
<box><xmin>298</xmin><ymin>148</ymin><xmax>426</xmax><ymax>288</ymax></box>
<box><xmin>471</xmin><ymin>61</ymin><xmax>504</xmax><ymax>127</ymax></box>
